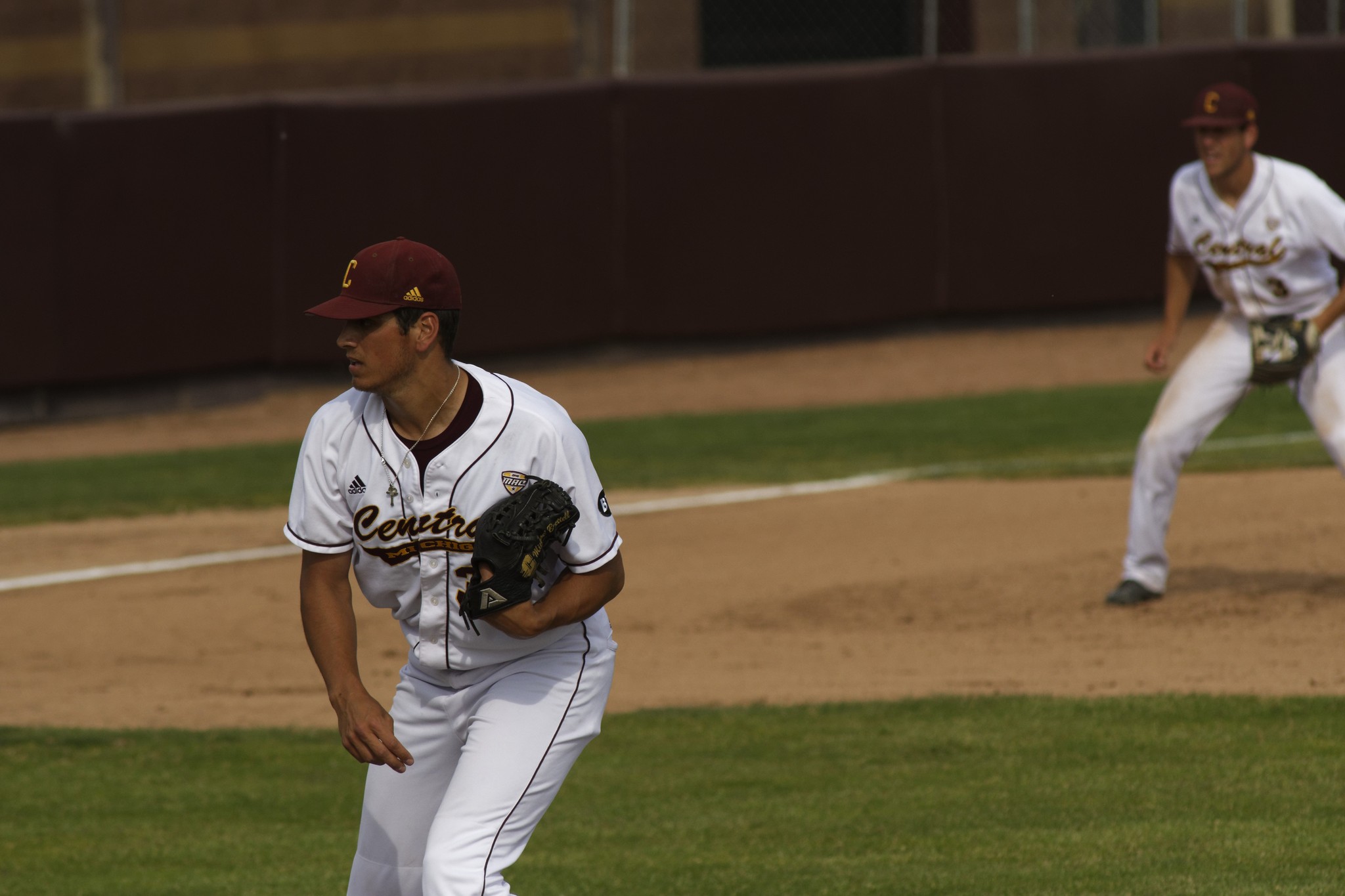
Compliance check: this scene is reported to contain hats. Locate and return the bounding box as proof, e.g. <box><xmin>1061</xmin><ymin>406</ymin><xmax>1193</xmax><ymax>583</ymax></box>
<box><xmin>1183</xmin><ymin>83</ymin><xmax>1257</xmax><ymax>129</ymax></box>
<box><xmin>301</xmin><ymin>235</ymin><xmax>461</xmax><ymax>322</ymax></box>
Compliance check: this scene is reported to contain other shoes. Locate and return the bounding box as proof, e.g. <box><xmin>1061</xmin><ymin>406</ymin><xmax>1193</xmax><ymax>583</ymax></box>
<box><xmin>1104</xmin><ymin>582</ymin><xmax>1159</xmax><ymax>602</ymax></box>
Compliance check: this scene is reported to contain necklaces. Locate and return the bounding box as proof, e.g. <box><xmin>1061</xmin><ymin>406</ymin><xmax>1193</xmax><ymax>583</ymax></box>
<box><xmin>381</xmin><ymin>365</ymin><xmax>460</xmax><ymax>507</ymax></box>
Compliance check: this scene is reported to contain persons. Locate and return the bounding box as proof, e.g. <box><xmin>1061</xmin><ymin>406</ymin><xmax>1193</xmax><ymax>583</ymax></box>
<box><xmin>1107</xmin><ymin>87</ymin><xmax>1344</xmax><ymax>608</ymax></box>
<box><xmin>284</xmin><ymin>240</ymin><xmax>626</xmax><ymax>895</ymax></box>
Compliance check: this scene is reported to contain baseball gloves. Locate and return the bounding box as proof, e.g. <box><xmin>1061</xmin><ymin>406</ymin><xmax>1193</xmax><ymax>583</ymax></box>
<box><xmin>1250</xmin><ymin>313</ymin><xmax>1320</xmax><ymax>384</ymax></box>
<box><xmin>460</xmin><ymin>480</ymin><xmax>580</xmax><ymax>620</ymax></box>
